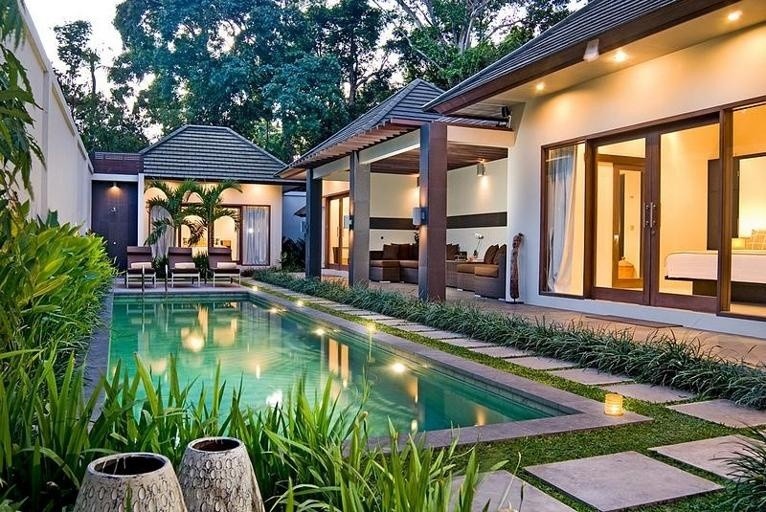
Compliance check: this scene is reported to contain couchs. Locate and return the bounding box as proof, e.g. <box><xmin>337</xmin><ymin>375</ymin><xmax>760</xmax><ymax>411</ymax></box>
<box><xmin>369</xmin><ymin>251</ymin><xmax>506</xmax><ymax>299</ymax></box>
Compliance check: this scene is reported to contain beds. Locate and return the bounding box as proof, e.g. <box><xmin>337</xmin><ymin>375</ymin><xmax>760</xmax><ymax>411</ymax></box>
<box><xmin>664</xmin><ymin>250</ymin><xmax>766</xmax><ymax>303</ymax></box>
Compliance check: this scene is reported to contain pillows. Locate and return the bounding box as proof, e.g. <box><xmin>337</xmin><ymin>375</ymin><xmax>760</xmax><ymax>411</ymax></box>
<box><xmin>175</xmin><ymin>262</ymin><xmax>196</xmax><ymax>269</ymax></box>
<box><xmin>382</xmin><ymin>243</ymin><xmax>507</xmax><ymax>265</ymax></box>
<box><xmin>217</xmin><ymin>262</ymin><xmax>237</xmax><ymax>269</ymax></box>
<box><xmin>130</xmin><ymin>262</ymin><xmax>152</xmax><ymax>269</ymax></box>
<box><xmin>732</xmin><ymin>229</ymin><xmax>766</xmax><ymax>250</ymax></box>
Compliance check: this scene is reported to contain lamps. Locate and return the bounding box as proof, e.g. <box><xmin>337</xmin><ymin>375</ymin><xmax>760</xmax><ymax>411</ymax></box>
<box><xmin>344</xmin><ymin>215</ymin><xmax>352</xmax><ymax>229</ymax></box>
<box><xmin>473</xmin><ymin>233</ymin><xmax>484</xmax><ymax>258</ymax></box>
<box><xmin>477</xmin><ymin>163</ymin><xmax>484</xmax><ymax>176</ymax></box>
<box><xmin>413</xmin><ymin>207</ymin><xmax>424</xmax><ymax>225</ymax></box>
<box><xmin>604</xmin><ymin>394</ymin><xmax>624</xmax><ymax>416</ymax></box>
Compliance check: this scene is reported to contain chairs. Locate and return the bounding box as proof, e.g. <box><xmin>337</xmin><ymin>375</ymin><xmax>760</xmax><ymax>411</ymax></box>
<box><xmin>125</xmin><ymin>246</ymin><xmax>156</xmax><ymax>288</ymax></box>
<box><xmin>204</xmin><ymin>247</ymin><xmax>241</xmax><ymax>287</ymax></box>
<box><xmin>165</xmin><ymin>247</ymin><xmax>201</xmax><ymax>289</ymax></box>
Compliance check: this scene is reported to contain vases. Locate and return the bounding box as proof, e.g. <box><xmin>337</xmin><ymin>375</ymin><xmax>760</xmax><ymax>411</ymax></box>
<box><xmin>73</xmin><ymin>452</ymin><xmax>188</xmax><ymax>512</ymax></box>
<box><xmin>176</xmin><ymin>437</ymin><xmax>265</xmax><ymax>512</ymax></box>
